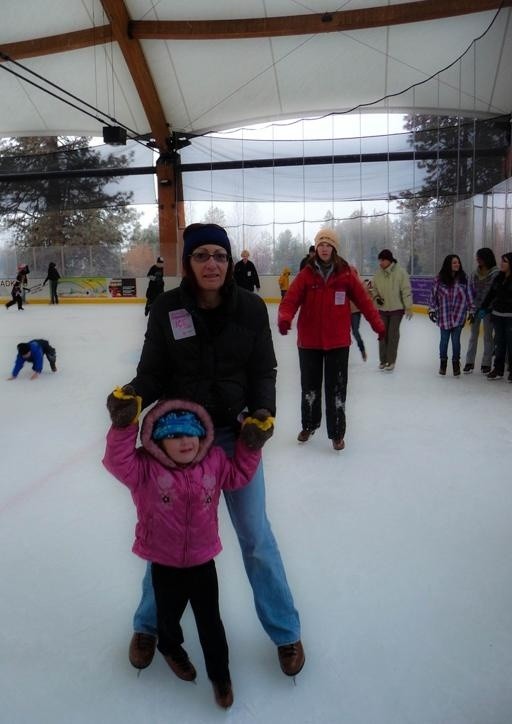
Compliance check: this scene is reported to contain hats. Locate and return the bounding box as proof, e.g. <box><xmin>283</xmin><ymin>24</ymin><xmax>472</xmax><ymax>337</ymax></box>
<box><xmin>378</xmin><ymin>248</ymin><xmax>393</xmax><ymax>261</ymax></box>
<box><xmin>18</xmin><ymin>343</ymin><xmax>31</xmax><ymax>356</ymax></box>
<box><xmin>314</xmin><ymin>229</ymin><xmax>338</xmax><ymax>251</ymax></box>
<box><xmin>153</xmin><ymin>408</ymin><xmax>205</xmax><ymax>439</ymax></box>
<box><xmin>157</xmin><ymin>256</ymin><xmax>164</xmax><ymax>263</ymax></box>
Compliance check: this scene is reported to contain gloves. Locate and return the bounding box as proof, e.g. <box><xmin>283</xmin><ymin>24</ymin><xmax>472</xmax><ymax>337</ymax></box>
<box><xmin>428</xmin><ymin>304</ymin><xmax>486</xmax><ymax>324</ymax></box>
<box><xmin>278</xmin><ymin>320</ymin><xmax>292</xmax><ymax>335</ymax></box>
<box><xmin>242</xmin><ymin>407</ymin><xmax>274</xmax><ymax>448</ymax></box>
<box><xmin>404</xmin><ymin>308</ymin><xmax>413</xmax><ymax>320</ymax></box>
<box><xmin>377</xmin><ymin>330</ymin><xmax>386</xmax><ymax>341</ymax></box>
<box><xmin>107</xmin><ymin>384</ymin><xmax>142</xmax><ymax>426</ymax></box>
<box><xmin>375</xmin><ymin>298</ymin><xmax>384</xmax><ymax>305</ymax></box>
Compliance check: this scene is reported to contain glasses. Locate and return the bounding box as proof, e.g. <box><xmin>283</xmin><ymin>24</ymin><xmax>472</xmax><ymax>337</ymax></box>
<box><xmin>188</xmin><ymin>252</ymin><xmax>231</xmax><ymax>262</ymax></box>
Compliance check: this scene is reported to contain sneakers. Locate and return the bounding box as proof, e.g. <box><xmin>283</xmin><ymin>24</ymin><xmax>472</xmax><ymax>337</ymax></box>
<box><xmin>160</xmin><ymin>653</ymin><xmax>197</xmax><ymax>680</ymax></box>
<box><xmin>333</xmin><ymin>438</ymin><xmax>345</xmax><ymax>450</ymax></box>
<box><xmin>50</xmin><ymin>361</ymin><xmax>57</xmax><ymax>371</ymax></box>
<box><xmin>129</xmin><ymin>632</ymin><xmax>157</xmax><ymax>669</ymax></box>
<box><xmin>212</xmin><ymin>679</ymin><xmax>234</xmax><ymax>707</ymax></box>
<box><xmin>297</xmin><ymin>429</ymin><xmax>310</xmax><ymax>441</ymax></box>
<box><xmin>277</xmin><ymin>639</ymin><xmax>305</xmax><ymax>675</ymax></box>
<box><xmin>379</xmin><ymin>359</ymin><xmax>511</xmax><ymax>382</ymax></box>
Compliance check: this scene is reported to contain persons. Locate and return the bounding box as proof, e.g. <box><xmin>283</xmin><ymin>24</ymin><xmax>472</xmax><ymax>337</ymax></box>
<box><xmin>463</xmin><ymin>248</ymin><xmax>501</xmax><ymax>372</ymax></box>
<box><xmin>349</xmin><ymin>266</ymin><xmax>368</xmax><ymax>361</ymax></box>
<box><xmin>278</xmin><ymin>228</ymin><xmax>386</xmax><ymax>450</ymax></box>
<box><xmin>147</xmin><ymin>257</ymin><xmax>165</xmax><ymax>311</ymax></box>
<box><xmin>102</xmin><ymin>385</ymin><xmax>274</xmax><ymax>710</ymax></box>
<box><xmin>16</xmin><ymin>264</ymin><xmax>30</xmax><ymax>303</ymax></box>
<box><xmin>279</xmin><ymin>266</ymin><xmax>291</xmax><ymax>299</ymax></box>
<box><xmin>428</xmin><ymin>254</ymin><xmax>478</xmax><ymax>376</ymax></box>
<box><xmin>42</xmin><ymin>262</ymin><xmax>60</xmax><ymax>304</ymax></box>
<box><xmin>126</xmin><ymin>223</ymin><xmax>306</xmax><ymax>676</ymax></box>
<box><xmin>368</xmin><ymin>249</ymin><xmax>413</xmax><ymax>370</ymax></box>
<box><xmin>5</xmin><ymin>276</ymin><xmax>30</xmax><ymax>310</ymax></box>
<box><xmin>481</xmin><ymin>252</ymin><xmax>512</xmax><ymax>382</ymax></box>
<box><xmin>8</xmin><ymin>338</ymin><xmax>57</xmax><ymax>380</ymax></box>
<box><xmin>300</xmin><ymin>246</ymin><xmax>316</xmax><ymax>272</ymax></box>
<box><xmin>234</xmin><ymin>250</ymin><xmax>260</xmax><ymax>293</ymax></box>
